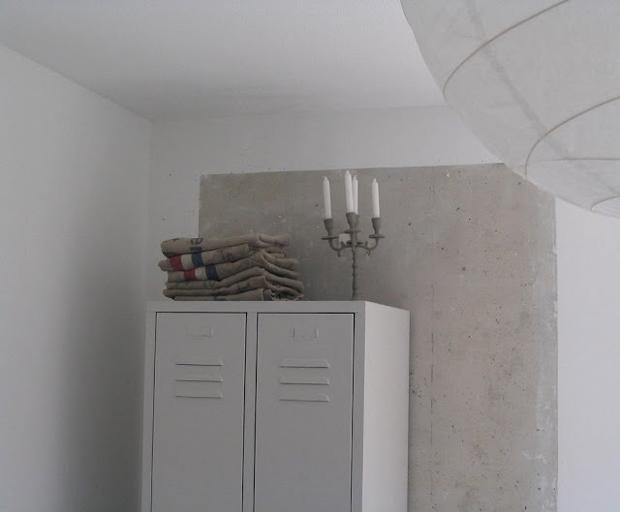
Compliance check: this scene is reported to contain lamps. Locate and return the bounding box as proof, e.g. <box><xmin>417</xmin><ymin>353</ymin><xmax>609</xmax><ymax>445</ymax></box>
<box><xmin>397</xmin><ymin>0</ymin><xmax>620</xmax><ymax>225</ymax></box>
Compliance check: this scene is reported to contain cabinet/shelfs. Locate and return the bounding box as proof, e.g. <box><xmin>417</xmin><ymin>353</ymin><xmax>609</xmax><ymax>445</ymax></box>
<box><xmin>137</xmin><ymin>296</ymin><xmax>410</xmax><ymax>512</ymax></box>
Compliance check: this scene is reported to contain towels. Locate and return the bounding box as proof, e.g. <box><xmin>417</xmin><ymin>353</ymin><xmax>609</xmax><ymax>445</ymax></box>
<box><xmin>158</xmin><ymin>230</ymin><xmax>305</xmax><ymax>302</ymax></box>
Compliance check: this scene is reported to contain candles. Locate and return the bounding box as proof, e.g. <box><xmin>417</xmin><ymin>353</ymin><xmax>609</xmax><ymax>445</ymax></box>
<box><xmin>322</xmin><ymin>174</ymin><xmax>331</xmax><ymax>220</ymax></box>
<box><xmin>370</xmin><ymin>176</ymin><xmax>380</xmax><ymax>218</ymax></box>
<box><xmin>351</xmin><ymin>174</ymin><xmax>359</xmax><ymax>214</ymax></box>
<box><xmin>344</xmin><ymin>169</ymin><xmax>354</xmax><ymax>212</ymax></box>
